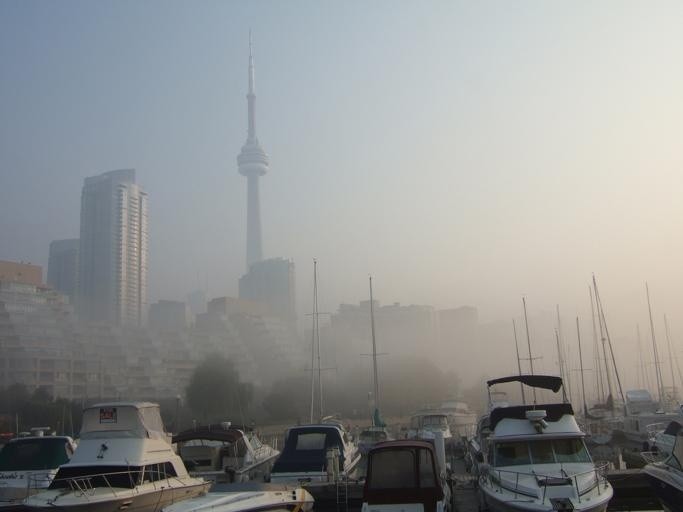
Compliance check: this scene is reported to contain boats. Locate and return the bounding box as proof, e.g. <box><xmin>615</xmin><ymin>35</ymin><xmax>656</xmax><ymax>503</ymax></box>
<box><xmin>161</xmin><ymin>482</ymin><xmax>317</xmax><ymax>512</ymax></box>
<box><xmin>268</xmin><ymin>421</ymin><xmax>364</xmax><ymax>494</ymax></box>
<box><xmin>20</xmin><ymin>401</ymin><xmax>215</xmax><ymax>511</ymax></box>
<box><xmin>168</xmin><ymin>419</ymin><xmax>281</xmax><ymax>487</ymax></box>
<box><xmin>469</xmin><ymin>375</ymin><xmax>615</xmax><ymax>512</ymax></box>
<box><xmin>301</xmin><ymin>260</ymin><xmax>477</xmax><ymax>451</ymax></box>
<box><xmin>0</xmin><ymin>435</ymin><xmax>78</xmax><ymax>511</ymax></box>
<box><xmin>358</xmin><ymin>437</ymin><xmax>453</xmax><ymax>512</ymax></box>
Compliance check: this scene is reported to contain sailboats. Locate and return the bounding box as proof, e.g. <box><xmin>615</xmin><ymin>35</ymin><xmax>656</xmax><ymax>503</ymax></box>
<box><xmin>511</xmin><ymin>259</ymin><xmax>683</xmax><ymax>512</ymax></box>
